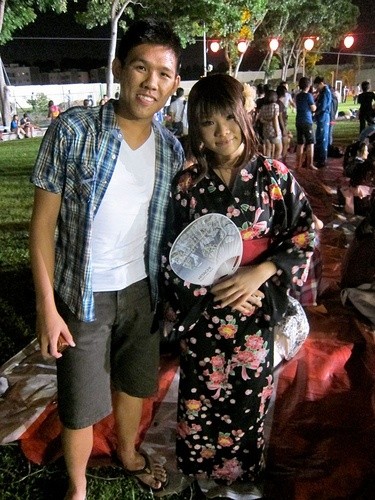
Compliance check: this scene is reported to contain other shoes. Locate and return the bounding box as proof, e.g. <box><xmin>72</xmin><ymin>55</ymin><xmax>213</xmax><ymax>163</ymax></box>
<box><xmin>316</xmin><ymin>160</ymin><xmax>325</xmax><ymax>168</ymax></box>
<box><xmin>17</xmin><ymin>135</ymin><xmax>20</xmax><ymax>139</ymax></box>
<box><xmin>25</xmin><ymin>134</ymin><xmax>28</xmax><ymax>138</ymax></box>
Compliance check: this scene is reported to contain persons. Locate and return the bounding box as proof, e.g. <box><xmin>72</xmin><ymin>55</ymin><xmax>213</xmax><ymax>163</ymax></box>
<box><xmin>157</xmin><ymin>74</ymin><xmax>316</xmax><ymax>500</ymax></box>
<box><xmin>48</xmin><ymin>100</ymin><xmax>61</xmax><ymax>123</ymax></box>
<box><xmin>10</xmin><ymin>114</ymin><xmax>33</xmax><ymax>138</ymax></box>
<box><xmin>29</xmin><ymin>21</ymin><xmax>186</xmax><ymax>500</ymax></box>
<box><xmin>354</xmin><ymin>83</ymin><xmax>360</xmax><ymax>104</ymax></box>
<box><xmin>342</xmin><ymin>141</ymin><xmax>375</xmax><ymax>187</ymax></box>
<box><xmin>169</xmin><ymin>87</ymin><xmax>188</xmax><ymax>136</ymax></box>
<box><xmin>358</xmin><ymin>81</ymin><xmax>375</xmax><ymax>133</ymax></box>
<box><xmin>253</xmin><ymin>77</ymin><xmax>338</xmax><ymax>170</ymax></box>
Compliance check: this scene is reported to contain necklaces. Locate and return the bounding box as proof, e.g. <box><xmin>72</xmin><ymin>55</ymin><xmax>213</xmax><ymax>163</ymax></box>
<box><xmin>216</xmin><ymin>165</ymin><xmax>227</xmax><ymax>186</ymax></box>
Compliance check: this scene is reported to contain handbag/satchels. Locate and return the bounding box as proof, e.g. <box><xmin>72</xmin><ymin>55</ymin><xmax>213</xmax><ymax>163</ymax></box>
<box><xmin>52</xmin><ymin>111</ymin><xmax>60</xmax><ymax>119</ymax></box>
<box><xmin>263</xmin><ymin>118</ymin><xmax>276</xmax><ymax>138</ymax></box>
<box><xmin>171</xmin><ymin>121</ymin><xmax>183</xmax><ymax>135</ymax></box>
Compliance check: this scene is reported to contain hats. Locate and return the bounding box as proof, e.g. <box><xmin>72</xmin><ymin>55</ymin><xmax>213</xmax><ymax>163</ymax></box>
<box><xmin>314</xmin><ymin>77</ymin><xmax>324</xmax><ymax>84</ymax></box>
<box><xmin>24</xmin><ymin>113</ymin><xmax>29</xmax><ymax>117</ymax></box>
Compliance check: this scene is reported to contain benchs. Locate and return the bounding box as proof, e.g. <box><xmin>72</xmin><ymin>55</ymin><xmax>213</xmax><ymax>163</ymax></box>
<box><xmin>1</xmin><ymin>131</ymin><xmax>39</xmax><ymax>140</ymax></box>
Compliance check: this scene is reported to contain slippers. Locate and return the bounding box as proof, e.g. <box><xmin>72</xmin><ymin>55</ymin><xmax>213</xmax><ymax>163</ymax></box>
<box><xmin>115</xmin><ymin>445</ymin><xmax>168</xmax><ymax>492</ymax></box>
